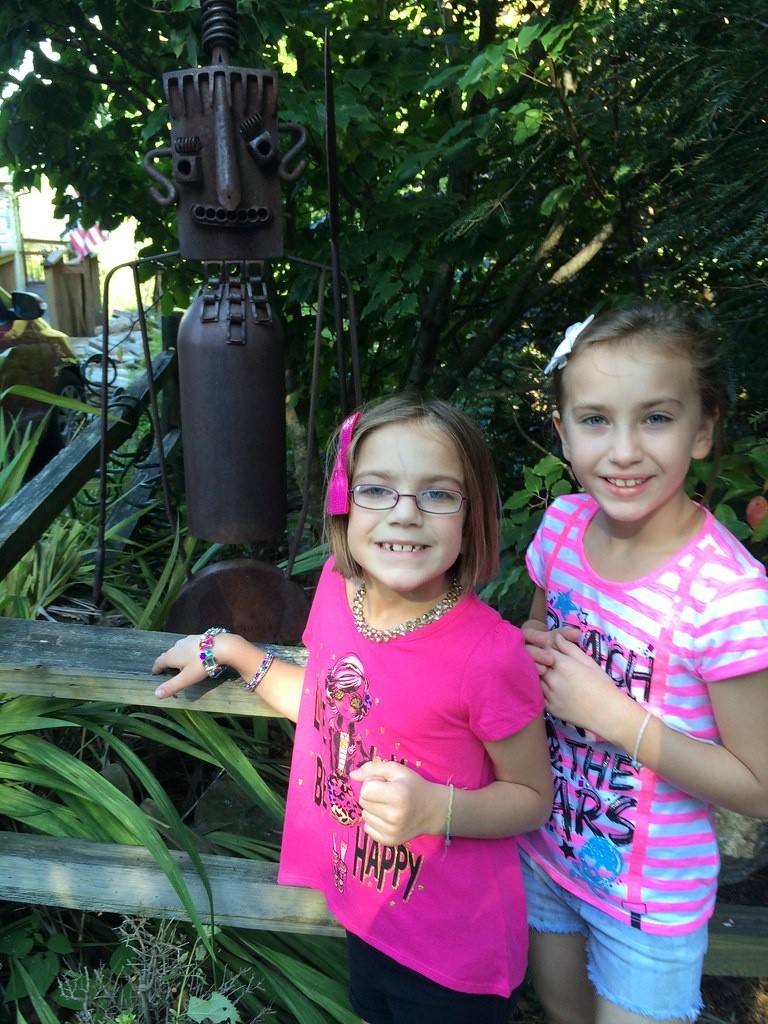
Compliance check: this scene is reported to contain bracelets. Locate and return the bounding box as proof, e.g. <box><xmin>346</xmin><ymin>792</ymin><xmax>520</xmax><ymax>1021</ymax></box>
<box><xmin>241</xmin><ymin>649</ymin><xmax>276</xmax><ymax>693</ymax></box>
<box><xmin>627</xmin><ymin>708</ymin><xmax>654</xmax><ymax>773</ymax></box>
<box><xmin>196</xmin><ymin>626</ymin><xmax>231</xmax><ymax>680</ymax></box>
<box><xmin>440</xmin><ymin>782</ymin><xmax>459</xmax><ymax>848</ymax></box>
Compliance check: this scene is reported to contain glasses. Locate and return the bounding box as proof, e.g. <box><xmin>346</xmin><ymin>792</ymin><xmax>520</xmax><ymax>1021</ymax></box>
<box><xmin>349</xmin><ymin>479</ymin><xmax>470</xmax><ymax>513</ymax></box>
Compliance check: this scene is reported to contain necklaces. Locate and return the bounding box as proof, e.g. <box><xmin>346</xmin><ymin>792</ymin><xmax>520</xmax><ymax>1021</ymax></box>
<box><xmin>351</xmin><ymin>575</ymin><xmax>466</xmax><ymax>642</ymax></box>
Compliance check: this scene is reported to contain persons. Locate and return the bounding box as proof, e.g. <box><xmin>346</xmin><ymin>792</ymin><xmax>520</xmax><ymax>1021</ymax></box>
<box><xmin>513</xmin><ymin>296</ymin><xmax>768</xmax><ymax>1024</ymax></box>
<box><xmin>99</xmin><ymin>0</ymin><xmax>361</xmax><ymax>552</ymax></box>
<box><xmin>146</xmin><ymin>389</ymin><xmax>556</xmax><ymax>1024</ymax></box>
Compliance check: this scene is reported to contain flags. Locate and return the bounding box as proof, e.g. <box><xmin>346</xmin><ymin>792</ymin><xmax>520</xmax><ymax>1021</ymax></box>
<box><xmin>66</xmin><ymin>217</ymin><xmax>111</xmax><ymax>263</ymax></box>
